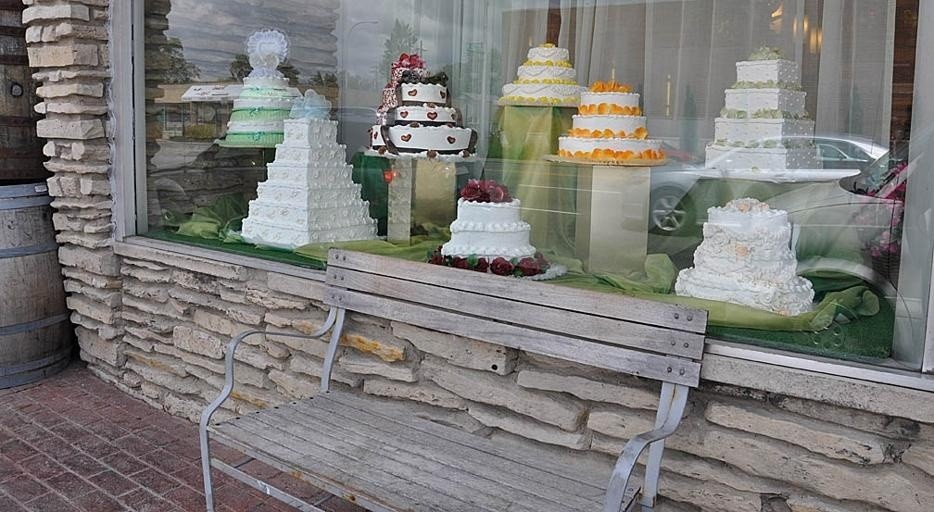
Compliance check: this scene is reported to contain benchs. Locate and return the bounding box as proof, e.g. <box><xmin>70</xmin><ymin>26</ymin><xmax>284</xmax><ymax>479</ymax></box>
<box><xmin>196</xmin><ymin>247</ymin><xmax>708</xmax><ymax>511</ymax></box>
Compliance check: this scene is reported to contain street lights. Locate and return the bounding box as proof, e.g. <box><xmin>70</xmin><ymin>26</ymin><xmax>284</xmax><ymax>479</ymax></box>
<box><xmin>341</xmin><ymin>22</ymin><xmax>378</xmax><ymax>141</ymax></box>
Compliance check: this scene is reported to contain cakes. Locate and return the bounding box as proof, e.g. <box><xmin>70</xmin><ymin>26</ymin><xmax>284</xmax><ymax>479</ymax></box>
<box><xmin>370</xmin><ymin>72</ymin><xmax>477</xmax><ymax>158</ymax></box>
<box><xmin>438</xmin><ymin>179</ymin><xmax>540</xmax><ymax>276</ymax></box>
<box><xmin>495</xmin><ymin>42</ymin><xmax>589</xmax><ymax>108</ymax></box>
<box><xmin>224</xmin><ymin>31</ymin><xmax>296</xmax><ymax>147</ymax></box>
<box><xmin>368</xmin><ymin>53</ymin><xmax>429</xmax><ymax>147</ymax></box>
<box><xmin>704</xmin><ymin>46</ymin><xmax>823</xmax><ymax>170</ymax></box>
<box><xmin>673</xmin><ymin>197</ymin><xmax>816</xmax><ymax>316</ymax></box>
<box><xmin>240</xmin><ymin>91</ymin><xmax>379</xmax><ymax>248</ymax></box>
<box><xmin>558</xmin><ymin>76</ymin><xmax>663</xmax><ymax>162</ymax></box>
